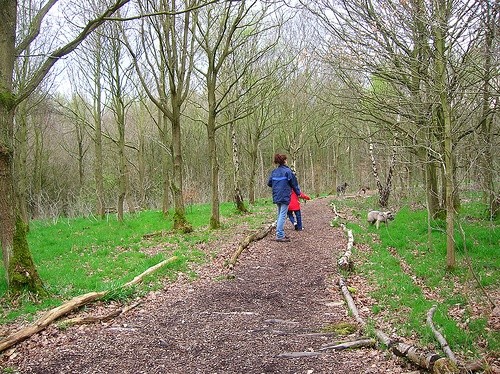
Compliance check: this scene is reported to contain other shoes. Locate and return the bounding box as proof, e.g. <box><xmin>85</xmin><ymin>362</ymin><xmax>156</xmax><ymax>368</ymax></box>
<box><xmin>293</xmin><ymin>225</ymin><xmax>298</xmax><ymax>230</ymax></box>
<box><xmin>276</xmin><ymin>236</ymin><xmax>291</xmax><ymax>242</ymax></box>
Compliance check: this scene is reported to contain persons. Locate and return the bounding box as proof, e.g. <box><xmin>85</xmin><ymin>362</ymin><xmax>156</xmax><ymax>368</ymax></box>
<box><xmin>267</xmin><ymin>153</ymin><xmax>301</xmax><ymax>242</ymax></box>
<box><xmin>287</xmin><ymin>187</ymin><xmax>311</xmax><ymax>230</ymax></box>
